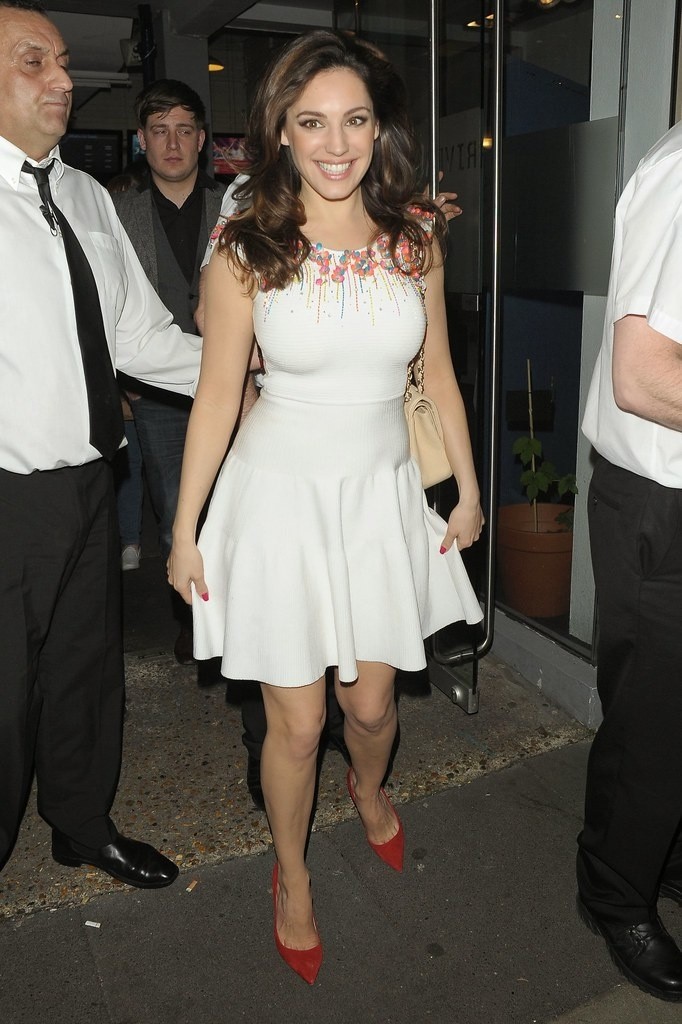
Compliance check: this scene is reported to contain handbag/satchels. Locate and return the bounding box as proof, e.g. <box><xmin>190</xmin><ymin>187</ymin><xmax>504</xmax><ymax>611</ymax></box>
<box><xmin>401</xmin><ymin>383</ymin><xmax>454</xmax><ymax>490</ymax></box>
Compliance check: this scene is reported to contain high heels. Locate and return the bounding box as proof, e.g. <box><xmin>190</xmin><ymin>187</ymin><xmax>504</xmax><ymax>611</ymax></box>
<box><xmin>346</xmin><ymin>767</ymin><xmax>405</xmax><ymax>873</ymax></box>
<box><xmin>273</xmin><ymin>862</ymin><xmax>323</xmax><ymax>985</ymax></box>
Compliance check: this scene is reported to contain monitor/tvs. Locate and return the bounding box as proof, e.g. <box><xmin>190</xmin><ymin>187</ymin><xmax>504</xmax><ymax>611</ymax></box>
<box><xmin>60</xmin><ymin>130</ymin><xmax>123</xmax><ymax>187</ymax></box>
<box><xmin>127</xmin><ymin>129</ymin><xmax>146</xmax><ymax>164</ymax></box>
<box><xmin>212</xmin><ymin>133</ymin><xmax>250</xmax><ymax>176</ymax></box>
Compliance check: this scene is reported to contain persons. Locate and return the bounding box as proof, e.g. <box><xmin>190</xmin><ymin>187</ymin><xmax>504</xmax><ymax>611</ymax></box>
<box><xmin>106</xmin><ymin>79</ymin><xmax>466</xmax><ymax>813</ymax></box>
<box><xmin>163</xmin><ymin>25</ymin><xmax>500</xmax><ymax>982</ymax></box>
<box><xmin>0</xmin><ymin>5</ymin><xmax>261</xmax><ymax>890</ymax></box>
<box><xmin>578</xmin><ymin>119</ymin><xmax>682</xmax><ymax>1001</ymax></box>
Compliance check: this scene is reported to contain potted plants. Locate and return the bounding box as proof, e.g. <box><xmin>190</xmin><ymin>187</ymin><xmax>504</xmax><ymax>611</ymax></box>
<box><xmin>496</xmin><ymin>436</ymin><xmax>577</xmax><ymax>621</ymax></box>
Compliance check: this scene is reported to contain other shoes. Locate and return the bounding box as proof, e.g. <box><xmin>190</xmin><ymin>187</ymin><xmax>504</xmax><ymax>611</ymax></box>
<box><xmin>246</xmin><ymin>755</ymin><xmax>269</xmax><ymax>812</ymax></box>
<box><xmin>173</xmin><ymin>616</ymin><xmax>198</xmax><ymax>666</ymax></box>
<box><xmin>658</xmin><ymin>874</ymin><xmax>682</xmax><ymax>907</ymax></box>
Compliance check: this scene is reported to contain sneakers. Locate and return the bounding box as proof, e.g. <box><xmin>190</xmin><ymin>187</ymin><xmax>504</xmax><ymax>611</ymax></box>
<box><xmin>121</xmin><ymin>543</ymin><xmax>144</xmax><ymax>571</ymax></box>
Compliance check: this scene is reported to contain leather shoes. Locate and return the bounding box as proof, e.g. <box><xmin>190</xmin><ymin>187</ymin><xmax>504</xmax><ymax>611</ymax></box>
<box><xmin>51</xmin><ymin>831</ymin><xmax>180</xmax><ymax>889</ymax></box>
<box><xmin>575</xmin><ymin>893</ymin><xmax>682</xmax><ymax>1002</ymax></box>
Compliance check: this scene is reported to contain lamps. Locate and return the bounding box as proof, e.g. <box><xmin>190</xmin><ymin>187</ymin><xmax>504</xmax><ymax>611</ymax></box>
<box><xmin>208</xmin><ymin>53</ymin><xmax>224</xmax><ymax>71</ymax></box>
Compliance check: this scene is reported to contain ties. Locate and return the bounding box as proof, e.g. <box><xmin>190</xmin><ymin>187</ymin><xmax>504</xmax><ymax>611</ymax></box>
<box><xmin>20</xmin><ymin>158</ymin><xmax>127</xmax><ymax>467</ymax></box>
